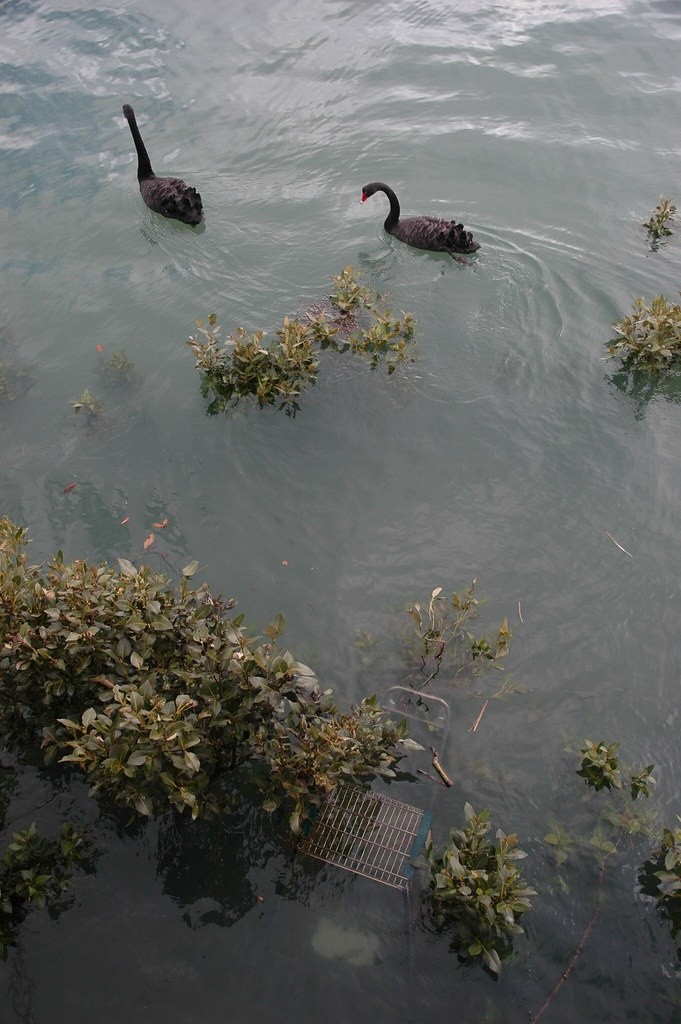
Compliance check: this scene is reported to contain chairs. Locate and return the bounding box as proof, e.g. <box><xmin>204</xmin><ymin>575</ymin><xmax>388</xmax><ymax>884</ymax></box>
<box><xmin>290</xmin><ymin>685</ymin><xmax>451</xmax><ymax>944</ymax></box>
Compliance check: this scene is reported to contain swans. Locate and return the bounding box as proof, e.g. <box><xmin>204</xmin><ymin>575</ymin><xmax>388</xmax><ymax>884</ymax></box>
<box><xmin>122</xmin><ymin>104</ymin><xmax>204</xmax><ymax>226</ymax></box>
<box><xmin>360</xmin><ymin>182</ymin><xmax>481</xmax><ymax>254</ymax></box>
